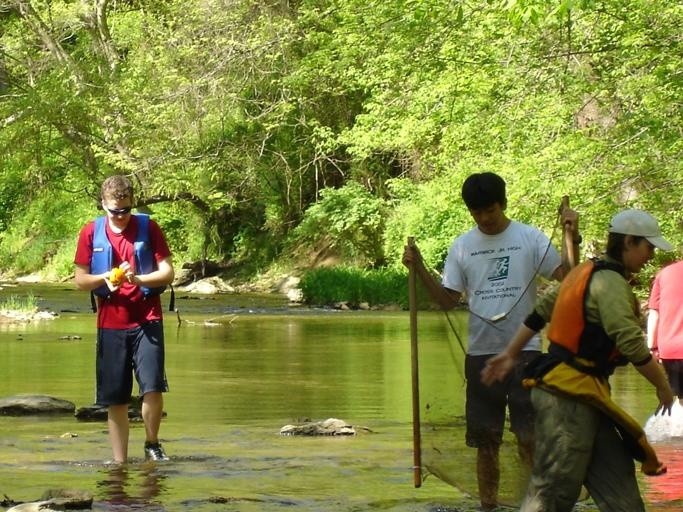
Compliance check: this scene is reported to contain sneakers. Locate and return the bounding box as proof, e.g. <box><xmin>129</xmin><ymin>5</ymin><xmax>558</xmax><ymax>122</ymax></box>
<box><xmin>141</xmin><ymin>440</ymin><xmax>171</xmax><ymax>462</ymax></box>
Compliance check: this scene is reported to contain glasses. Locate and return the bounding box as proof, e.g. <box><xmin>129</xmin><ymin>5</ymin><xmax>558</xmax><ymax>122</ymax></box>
<box><xmin>98</xmin><ymin>200</ymin><xmax>134</xmax><ymax>216</ymax></box>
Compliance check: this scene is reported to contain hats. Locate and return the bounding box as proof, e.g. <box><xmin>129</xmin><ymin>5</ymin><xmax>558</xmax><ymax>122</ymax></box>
<box><xmin>606</xmin><ymin>205</ymin><xmax>678</xmax><ymax>255</ymax></box>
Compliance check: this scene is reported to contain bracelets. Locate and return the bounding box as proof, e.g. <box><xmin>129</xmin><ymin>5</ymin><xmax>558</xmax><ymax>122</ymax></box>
<box><xmin>571</xmin><ymin>233</ymin><xmax>583</xmax><ymax>246</ymax></box>
<box><xmin>648</xmin><ymin>346</ymin><xmax>658</xmax><ymax>352</ymax></box>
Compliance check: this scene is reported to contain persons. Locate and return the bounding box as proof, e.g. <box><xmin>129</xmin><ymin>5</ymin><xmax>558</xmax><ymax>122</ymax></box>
<box><xmin>401</xmin><ymin>172</ymin><xmax>582</xmax><ymax>509</ymax></box>
<box><xmin>94</xmin><ymin>461</ymin><xmax>167</xmax><ymax>511</ymax></box>
<box><xmin>71</xmin><ymin>174</ymin><xmax>175</xmax><ymax>470</ymax></box>
<box><xmin>646</xmin><ymin>255</ymin><xmax>682</xmax><ymax>410</ymax></box>
<box><xmin>479</xmin><ymin>209</ymin><xmax>678</xmax><ymax>512</ymax></box>
